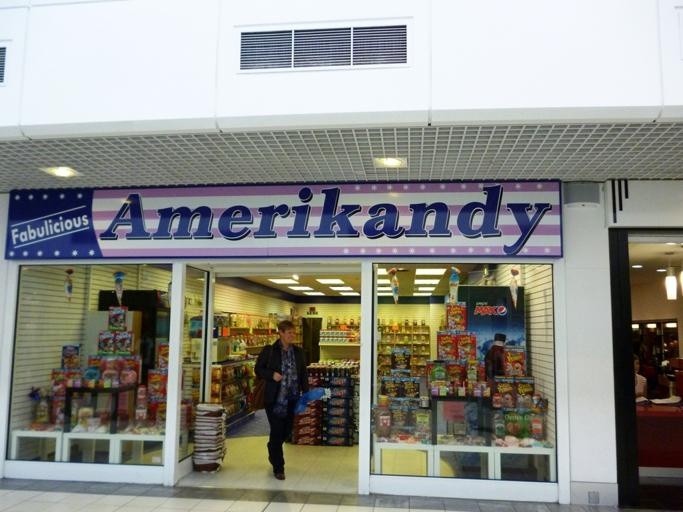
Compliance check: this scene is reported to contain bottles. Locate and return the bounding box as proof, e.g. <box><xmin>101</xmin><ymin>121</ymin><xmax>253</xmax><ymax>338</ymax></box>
<box><xmin>485</xmin><ymin>333</ymin><xmax>505</xmax><ymax>382</ymax></box>
<box><xmin>306</xmin><ymin>365</ymin><xmax>349</xmax><ymax>378</ymax></box>
<box><xmin>377</xmin><ymin>405</ymin><xmax>392</xmax><ymax>438</ymax></box>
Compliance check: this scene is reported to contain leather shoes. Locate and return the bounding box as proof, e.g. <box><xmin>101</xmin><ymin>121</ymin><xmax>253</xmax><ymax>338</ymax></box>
<box><xmin>274</xmin><ymin>472</ymin><xmax>285</xmax><ymax>480</ymax></box>
<box><xmin>268</xmin><ymin>455</ymin><xmax>272</xmax><ymax>464</ymax></box>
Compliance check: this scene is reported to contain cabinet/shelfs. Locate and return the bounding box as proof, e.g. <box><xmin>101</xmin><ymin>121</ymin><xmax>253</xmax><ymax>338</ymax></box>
<box><xmin>192</xmin><ymin>359</ymin><xmax>255</xmax><ymax>434</ymax></box>
<box><xmin>379</xmin><ymin>324</ymin><xmax>430</xmax><ymax>367</ymax></box>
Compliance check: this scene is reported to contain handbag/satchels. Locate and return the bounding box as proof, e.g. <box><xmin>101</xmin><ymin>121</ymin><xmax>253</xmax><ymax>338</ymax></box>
<box><xmin>251</xmin><ymin>345</ymin><xmax>273</xmax><ymax>410</ymax></box>
<box><xmin>636</xmin><ymin>396</ymin><xmax>650</xmax><ymax>405</ymax></box>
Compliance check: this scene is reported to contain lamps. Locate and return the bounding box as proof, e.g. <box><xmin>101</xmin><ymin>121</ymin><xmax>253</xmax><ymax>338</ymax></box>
<box><xmin>664</xmin><ymin>253</ymin><xmax>677</xmax><ymax>300</ymax></box>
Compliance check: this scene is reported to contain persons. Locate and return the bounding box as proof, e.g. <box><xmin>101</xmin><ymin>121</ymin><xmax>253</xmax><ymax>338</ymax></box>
<box><xmin>255</xmin><ymin>319</ymin><xmax>309</xmax><ymax>480</ymax></box>
<box><xmin>632</xmin><ymin>354</ymin><xmax>648</xmax><ymax>397</ymax></box>
